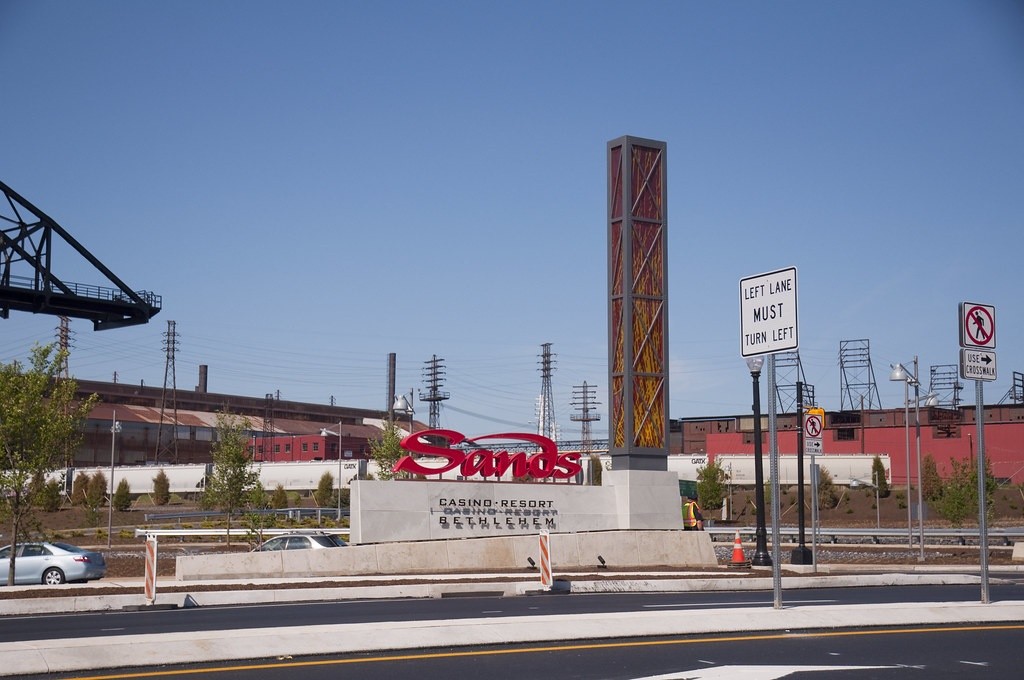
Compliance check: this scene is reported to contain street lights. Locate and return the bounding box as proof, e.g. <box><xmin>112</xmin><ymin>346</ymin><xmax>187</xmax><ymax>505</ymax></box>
<box><xmin>904</xmin><ymin>378</ymin><xmax>940</xmax><ymax>549</ymax></box>
<box><xmin>392</xmin><ymin>386</ymin><xmax>415</xmax><ymax>480</ymax></box>
<box><xmin>105</xmin><ymin>408</ymin><xmax>122</xmax><ymax>548</ymax></box>
<box><xmin>319</xmin><ymin>421</ymin><xmax>342</xmax><ymax>521</ymax></box>
<box><xmin>746</xmin><ymin>355</ymin><xmax>774</xmax><ymax>566</ymax></box>
<box><xmin>889</xmin><ymin>354</ymin><xmax>924</xmax><ymax>563</ymax></box>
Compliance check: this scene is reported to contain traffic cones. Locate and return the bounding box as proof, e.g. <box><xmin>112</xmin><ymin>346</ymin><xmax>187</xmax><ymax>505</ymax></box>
<box><xmin>727</xmin><ymin>530</ymin><xmax>751</xmax><ymax>569</ymax></box>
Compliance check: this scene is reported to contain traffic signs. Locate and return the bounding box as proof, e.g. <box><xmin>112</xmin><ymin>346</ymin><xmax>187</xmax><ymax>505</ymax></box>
<box><xmin>962</xmin><ymin>348</ymin><xmax>998</xmax><ymax>382</ymax></box>
<box><xmin>804</xmin><ymin>437</ymin><xmax>821</xmax><ymax>456</ymax></box>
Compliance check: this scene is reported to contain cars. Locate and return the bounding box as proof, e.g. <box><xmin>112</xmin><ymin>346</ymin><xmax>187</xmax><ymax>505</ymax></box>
<box><xmin>0</xmin><ymin>541</ymin><xmax>108</xmax><ymax>587</ymax></box>
<box><xmin>249</xmin><ymin>529</ymin><xmax>348</xmax><ymax>552</ymax></box>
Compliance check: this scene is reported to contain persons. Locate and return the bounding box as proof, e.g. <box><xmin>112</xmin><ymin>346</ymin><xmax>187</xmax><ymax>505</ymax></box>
<box><xmin>682</xmin><ymin>491</ymin><xmax>704</xmax><ymax>531</ymax></box>
